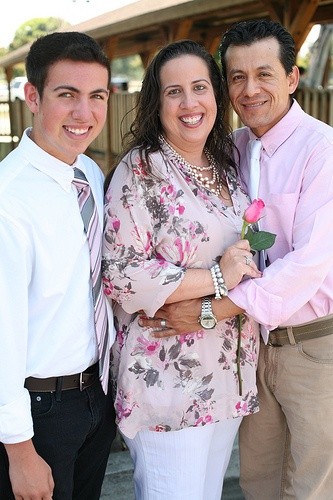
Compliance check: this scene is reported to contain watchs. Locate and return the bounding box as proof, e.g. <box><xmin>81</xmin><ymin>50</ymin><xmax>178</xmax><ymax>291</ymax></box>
<box><xmin>198</xmin><ymin>297</ymin><xmax>218</xmax><ymax>330</ymax></box>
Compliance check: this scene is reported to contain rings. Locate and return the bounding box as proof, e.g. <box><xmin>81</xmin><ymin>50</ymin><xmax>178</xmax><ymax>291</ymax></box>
<box><xmin>244</xmin><ymin>255</ymin><xmax>252</xmax><ymax>266</ymax></box>
<box><xmin>161</xmin><ymin>319</ymin><xmax>166</xmax><ymax>328</ymax></box>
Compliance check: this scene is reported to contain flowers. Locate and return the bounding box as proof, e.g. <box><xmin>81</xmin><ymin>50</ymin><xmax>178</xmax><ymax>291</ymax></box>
<box><xmin>237</xmin><ymin>199</ymin><xmax>277</xmax><ymax>396</ymax></box>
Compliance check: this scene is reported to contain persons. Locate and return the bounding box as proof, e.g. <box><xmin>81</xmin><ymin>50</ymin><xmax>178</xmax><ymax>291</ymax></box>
<box><xmin>138</xmin><ymin>16</ymin><xmax>333</xmax><ymax>500</ymax></box>
<box><xmin>100</xmin><ymin>40</ymin><xmax>263</xmax><ymax>500</ymax></box>
<box><xmin>0</xmin><ymin>31</ymin><xmax>117</xmax><ymax>499</ymax></box>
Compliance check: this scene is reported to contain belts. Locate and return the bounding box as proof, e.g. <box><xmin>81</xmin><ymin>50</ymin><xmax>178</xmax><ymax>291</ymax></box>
<box><xmin>260</xmin><ymin>318</ymin><xmax>333</xmax><ymax>347</ymax></box>
<box><xmin>25</xmin><ymin>373</ymin><xmax>96</xmax><ymax>393</ymax></box>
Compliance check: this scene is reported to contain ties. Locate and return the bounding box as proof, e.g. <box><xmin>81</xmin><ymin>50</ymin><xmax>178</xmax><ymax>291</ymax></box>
<box><xmin>248</xmin><ymin>140</ymin><xmax>266</xmax><ymax>273</ymax></box>
<box><xmin>72</xmin><ymin>166</ymin><xmax>109</xmax><ymax>395</ymax></box>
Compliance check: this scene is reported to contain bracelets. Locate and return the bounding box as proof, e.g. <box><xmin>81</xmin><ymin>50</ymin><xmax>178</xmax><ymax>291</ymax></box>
<box><xmin>209</xmin><ymin>266</ymin><xmax>222</xmax><ymax>300</ymax></box>
<box><xmin>214</xmin><ymin>263</ymin><xmax>229</xmax><ymax>297</ymax></box>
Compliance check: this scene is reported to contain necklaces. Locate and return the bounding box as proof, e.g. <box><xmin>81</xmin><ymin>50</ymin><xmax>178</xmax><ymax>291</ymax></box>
<box><xmin>159</xmin><ymin>134</ymin><xmax>223</xmax><ymax>198</ymax></box>
